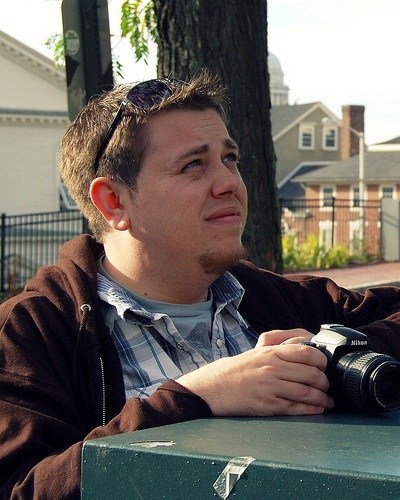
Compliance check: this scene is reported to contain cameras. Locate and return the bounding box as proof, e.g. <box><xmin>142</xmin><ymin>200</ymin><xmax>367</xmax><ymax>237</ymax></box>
<box><xmin>301</xmin><ymin>323</ymin><xmax>400</xmax><ymax>413</ymax></box>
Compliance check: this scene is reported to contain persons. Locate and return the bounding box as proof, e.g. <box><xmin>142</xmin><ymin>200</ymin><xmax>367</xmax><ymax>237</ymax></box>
<box><xmin>0</xmin><ymin>70</ymin><xmax>400</xmax><ymax>500</ymax></box>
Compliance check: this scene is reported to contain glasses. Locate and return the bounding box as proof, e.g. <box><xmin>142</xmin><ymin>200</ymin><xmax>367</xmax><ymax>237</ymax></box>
<box><xmin>90</xmin><ymin>76</ymin><xmax>190</xmax><ymax>175</ymax></box>
